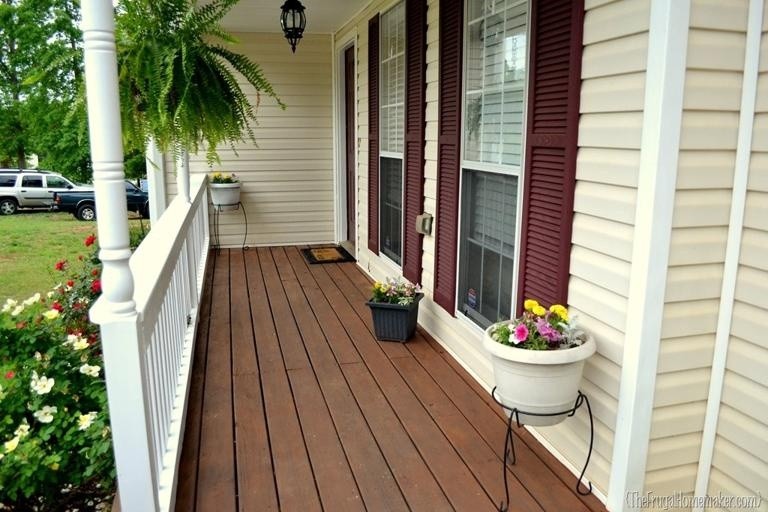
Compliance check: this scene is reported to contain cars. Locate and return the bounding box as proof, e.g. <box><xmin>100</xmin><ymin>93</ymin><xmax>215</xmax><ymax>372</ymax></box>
<box><xmin>0</xmin><ymin>168</ymin><xmax>76</xmax><ymax>215</ymax></box>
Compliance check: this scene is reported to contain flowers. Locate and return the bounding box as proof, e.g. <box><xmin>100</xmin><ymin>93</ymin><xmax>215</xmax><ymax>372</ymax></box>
<box><xmin>495</xmin><ymin>298</ymin><xmax>583</xmax><ymax>350</ymax></box>
<box><xmin>372</xmin><ymin>275</ymin><xmax>421</xmax><ymax>308</ymax></box>
<box><xmin>209</xmin><ymin>170</ymin><xmax>240</xmax><ymax>184</ymax></box>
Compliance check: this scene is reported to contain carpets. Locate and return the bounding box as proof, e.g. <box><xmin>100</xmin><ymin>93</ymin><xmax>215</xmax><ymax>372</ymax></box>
<box><xmin>300</xmin><ymin>246</ymin><xmax>357</xmax><ymax>265</ymax></box>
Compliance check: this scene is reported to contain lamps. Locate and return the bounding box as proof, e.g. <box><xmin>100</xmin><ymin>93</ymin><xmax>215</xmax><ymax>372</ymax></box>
<box><xmin>279</xmin><ymin>0</ymin><xmax>308</xmax><ymax>56</ymax></box>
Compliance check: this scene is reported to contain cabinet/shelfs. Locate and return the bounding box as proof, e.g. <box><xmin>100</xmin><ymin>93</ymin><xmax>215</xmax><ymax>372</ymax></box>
<box><xmin>208</xmin><ymin>202</ymin><xmax>250</xmax><ymax>256</ymax></box>
<box><xmin>490</xmin><ymin>387</ymin><xmax>594</xmax><ymax>512</ymax></box>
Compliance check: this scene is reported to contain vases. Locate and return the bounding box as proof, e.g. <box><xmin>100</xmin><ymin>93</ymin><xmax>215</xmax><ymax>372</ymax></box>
<box><xmin>365</xmin><ymin>293</ymin><xmax>424</xmax><ymax>343</ymax></box>
<box><xmin>206</xmin><ymin>183</ymin><xmax>240</xmax><ymax>211</ymax></box>
<box><xmin>484</xmin><ymin>317</ymin><xmax>596</xmax><ymax>426</ymax></box>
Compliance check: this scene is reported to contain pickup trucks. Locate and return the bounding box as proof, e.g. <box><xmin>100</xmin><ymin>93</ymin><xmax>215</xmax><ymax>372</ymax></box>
<box><xmin>54</xmin><ymin>181</ymin><xmax>151</xmax><ymax>221</ymax></box>
